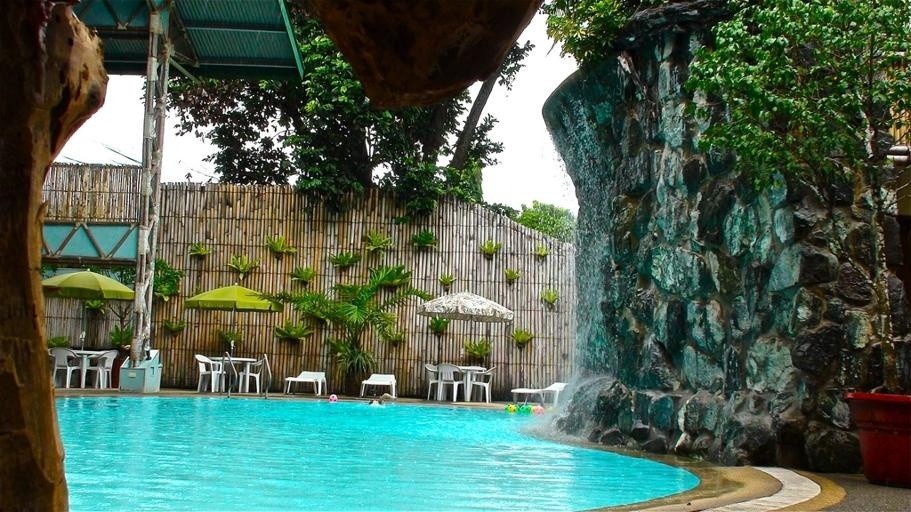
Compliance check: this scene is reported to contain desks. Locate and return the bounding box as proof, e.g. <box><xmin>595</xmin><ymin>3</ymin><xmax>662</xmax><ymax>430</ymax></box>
<box><xmin>71</xmin><ymin>350</ymin><xmax>102</xmax><ymax>388</ymax></box>
<box><xmin>457</xmin><ymin>366</ymin><xmax>487</xmax><ymax>372</ymax></box>
<box><xmin>209</xmin><ymin>357</ymin><xmax>257</xmax><ymax>392</ymax></box>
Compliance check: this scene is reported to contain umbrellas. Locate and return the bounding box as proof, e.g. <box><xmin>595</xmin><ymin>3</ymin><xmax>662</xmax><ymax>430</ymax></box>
<box><xmin>41</xmin><ymin>266</ymin><xmax>135</xmax><ymax>351</ymax></box>
<box><xmin>416</xmin><ymin>290</ymin><xmax>515</xmax><ymax>366</ymax></box>
<box><xmin>184</xmin><ymin>281</ymin><xmax>283</xmax><ymax>357</ymax></box>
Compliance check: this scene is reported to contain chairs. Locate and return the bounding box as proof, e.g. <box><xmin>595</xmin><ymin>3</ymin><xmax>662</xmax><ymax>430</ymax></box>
<box><xmin>50</xmin><ymin>347</ymin><xmax>81</xmax><ymax>388</ymax></box>
<box><xmin>467</xmin><ymin>366</ymin><xmax>497</xmax><ymax>403</ymax></box>
<box><xmin>237</xmin><ymin>360</ymin><xmax>263</xmax><ymax>393</ymax></box>
<box><xmin>360</xmin><ymin>374</ymin><xmax>396</xmax><ymax>398</ymax></box>
<box><xmin>425</xmin><ymin>364</ymin><xmax>438</xmax><ymax>400</ymax></box>
<box><xmin>511</xmin><ymin>383</ymin><xmax>568</xmax><ymax>408</ymax></box>
<box><xmin>285</xmin><ymin>371</ymin><xmax>327</xmax><ymax>395</ymax></box>
<box><xmin>87</xmin><ymin>350</ymin><xmax>118</xmax><ymax>389</ymax></box>
<box><xmin>195</xmin><ymin>354</ymin><xmax>226</xmax><ymax>392</ymax></box>
<box><xmin>437</xmin><ymin>364</ymin><xmax>467</xmax><ymax>403</ymax></box>
